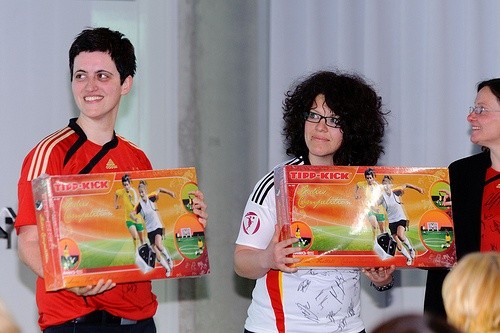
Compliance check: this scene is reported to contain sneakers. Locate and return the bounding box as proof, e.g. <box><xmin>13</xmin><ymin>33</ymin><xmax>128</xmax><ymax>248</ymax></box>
<box><xmin>407</xmin><ymin>250</ymin><xmax>416</xmax><ymax>266</ymax></box>
<box><xmin>164</xmin><ymin>261</ymin><xmax>173</xmax><ymax>277</ymax></box>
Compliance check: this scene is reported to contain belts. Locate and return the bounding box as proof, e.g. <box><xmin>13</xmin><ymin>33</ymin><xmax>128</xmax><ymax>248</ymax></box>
<box><xmin>66</xmin><ymin>312</ymin><xmax>121</xmax><ymax>325</ymax></box>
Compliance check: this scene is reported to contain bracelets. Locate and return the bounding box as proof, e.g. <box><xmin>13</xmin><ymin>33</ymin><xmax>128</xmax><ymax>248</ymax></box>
<box><xmin>371</xmin><ymin>281</ymin><xmax>394</xmax><ymax>292</ymax></box>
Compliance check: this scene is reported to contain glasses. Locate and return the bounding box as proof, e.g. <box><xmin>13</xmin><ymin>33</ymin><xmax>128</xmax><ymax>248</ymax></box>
<box><xmin>469</xmin><ymin>107</ymin><xmax>500</xmax><ymax>114</ymax></box>
<box><xmin>303</xmin><ymin>111</ymin><xmax>341</xmax><ymax>128</ymax></box>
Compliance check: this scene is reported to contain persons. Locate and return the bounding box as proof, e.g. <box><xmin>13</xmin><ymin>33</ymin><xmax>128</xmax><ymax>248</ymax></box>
<box><xmin>440</xmin><ymin>253</ymin><xmax>500</xmax><ymax>332</ymax></box>
<box><xmin>16</xmin><ymin>27</ymin><xmax>206</xmax><ymax>333</ymax></box>
<box><xmin>355</xmin><ymin>167</ymin><xmax>425</xmax><ymax>266</ymax></box>
<box><xmin>233</xmin><ymin>71</ymin><xmax>396</xmax><ymax>333</ymax></box>
<box><xmin>423</xmin><ymin>79</ymin><xmax>500</xmax><ymax>314</ymax></box>
<box><xmin>114</xmin><ymin>175</ymin><xmax>175</xmax><ymax>277</ymax></box>
